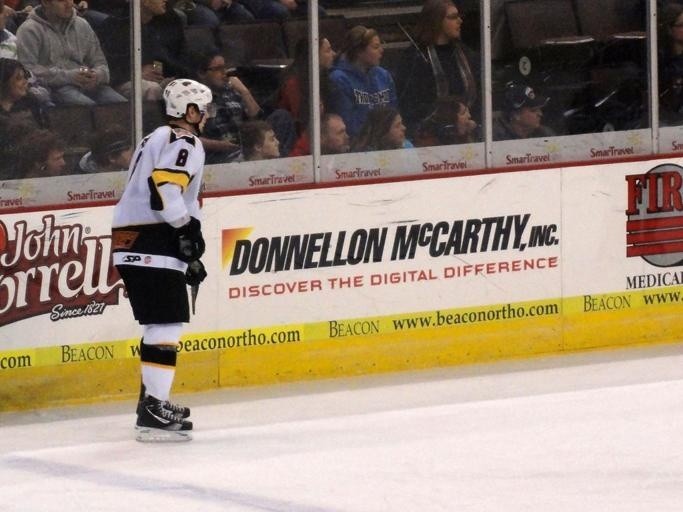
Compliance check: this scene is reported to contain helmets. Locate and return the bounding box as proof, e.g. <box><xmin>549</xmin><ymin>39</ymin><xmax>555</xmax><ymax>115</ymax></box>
<box><xmin>161</xmin><ymin>77</ymin><xmax>218</xmax><ymax>119</ymax></box>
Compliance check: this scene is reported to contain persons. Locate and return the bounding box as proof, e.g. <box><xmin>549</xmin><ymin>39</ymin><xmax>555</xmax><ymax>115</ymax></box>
<box><xmin>111</xmin><ymin>79</ymin><xmax>213</xmax><ymax>432</ymax></box>
<box><xmin>1</xmin><ymin>0</ymin><xmax>682</xmax><ymax>179</ymax></box>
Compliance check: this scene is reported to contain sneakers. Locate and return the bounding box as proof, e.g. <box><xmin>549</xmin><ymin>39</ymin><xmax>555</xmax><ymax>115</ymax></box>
<box><xmin>128</xmin><ymin>386</ymin><xmax>190</xmax><ymax>418</ymax></box>
<box><xmin>132</xmin><ymin>395</ymin><xmax>193</xmax><ymax>436</ymax></box>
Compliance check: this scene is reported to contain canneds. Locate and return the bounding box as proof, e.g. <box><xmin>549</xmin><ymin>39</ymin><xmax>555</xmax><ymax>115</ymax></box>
<box><xmin>151</xmin><ymin>61</ymin><xmax>162</xmax><ymax>72</ymax></box>
<box><xmin>80</xmin><ymin>65</ymin><xmax>89</xmax><ymax>77</ymax></box>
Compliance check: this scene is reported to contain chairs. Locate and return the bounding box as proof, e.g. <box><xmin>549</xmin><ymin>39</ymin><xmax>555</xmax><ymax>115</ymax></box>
<box><xmin>38</xmin><ymin>12</ymin><xmax>352</xmax><ymax>151</ymax></box>
<box><xmin>502</xmin><ymin>0</ymin><xmax>646</xmax><ymax>90</ymax></box>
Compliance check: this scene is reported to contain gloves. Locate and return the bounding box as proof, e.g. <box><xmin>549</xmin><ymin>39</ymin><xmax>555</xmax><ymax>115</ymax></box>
<box><xmin>185</xmin><ymin>259</ymin><xmax>207</xmax><ymax>288</ymax></box>
<box><xmin>175</xmin><ymin>216</ymin><xmax>205</xmax><ymax>263</ymax></box>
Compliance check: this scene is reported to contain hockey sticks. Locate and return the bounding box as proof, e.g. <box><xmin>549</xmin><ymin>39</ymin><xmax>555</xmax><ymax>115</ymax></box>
<box><xmin>183</xmin><ymin>245</ymin><xmax>199</xmax><ymax>315</ymax></box>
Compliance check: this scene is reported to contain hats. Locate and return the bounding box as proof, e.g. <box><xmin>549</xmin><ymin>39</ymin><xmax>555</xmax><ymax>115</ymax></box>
<box><xmin>498</xmin><ymin>80</ymin><xmax>551</xmax><ymax>111</ymax></box>
